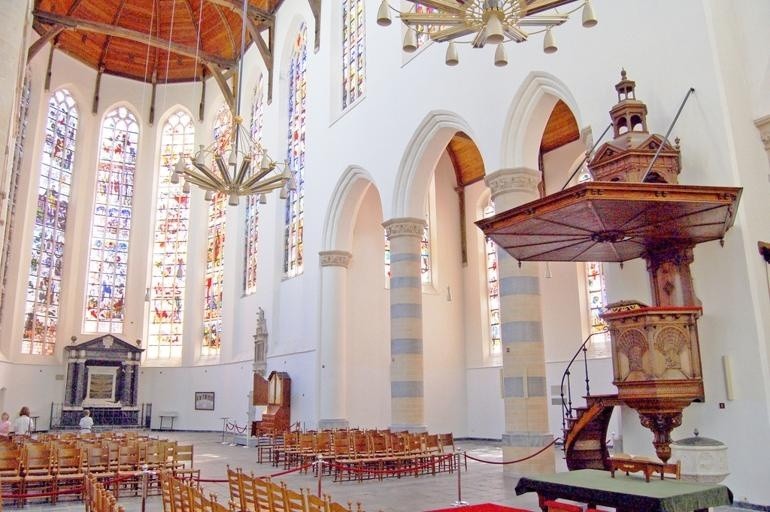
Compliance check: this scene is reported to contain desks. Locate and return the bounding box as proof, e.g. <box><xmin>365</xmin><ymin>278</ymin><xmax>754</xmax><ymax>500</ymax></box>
<box><xmin>158</xmin><ymin>415</ymin><xmax>178</xmax><ymax>431</ymax></box>
<box><xmin>514</xmin><ymin>466</ymin><xmax>734</xmax><ymax>512</ymax></box>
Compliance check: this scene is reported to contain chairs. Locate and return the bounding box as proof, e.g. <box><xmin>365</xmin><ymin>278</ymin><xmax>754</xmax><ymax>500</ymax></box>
<box><xmin>0</xmin><ymin>429</ymin><xmax>198</xmax><ymax>503</ymax></box>
<box><xmin>81</xmin><ymin>466</ymin><xmax>362</xmax><ymax>511</ymax></box>
<box><xmin>253</xmin><ymin>425</ymin><xmax>467</xmax><ymax>481</ymax></box>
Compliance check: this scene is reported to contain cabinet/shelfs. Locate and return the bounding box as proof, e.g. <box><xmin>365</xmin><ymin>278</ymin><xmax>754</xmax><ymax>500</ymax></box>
<box><xmin>250</xmin><ymin>370</ymin><xmax>291</xmax><ymax>438</ymax></box>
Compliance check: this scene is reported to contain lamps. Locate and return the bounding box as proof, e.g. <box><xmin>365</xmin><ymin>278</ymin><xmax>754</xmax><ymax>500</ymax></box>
<box><xmin>376</xmin><ymin>0</ymin><xmax>599</xmax><ymax>69</ymax></box>
<box><xmin>171</xmin><ymin>115</ymin><xmax>298</xmax><ymax>208</ymax></box>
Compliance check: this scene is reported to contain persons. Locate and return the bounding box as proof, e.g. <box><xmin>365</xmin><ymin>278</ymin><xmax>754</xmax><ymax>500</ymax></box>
<box><xmin>79</xmin><ymin>409</ymin><xmax>94</xmax><ymax>434</ymax></box>
<box><xmin>13</xmin><ymin>406</ymin><xmax>34</xmax><ymax>438</ymax></box>
<box><xmin>0</xmin><ymin>411</ymin><xmax>10</xmax><ymax>436</ymax></box>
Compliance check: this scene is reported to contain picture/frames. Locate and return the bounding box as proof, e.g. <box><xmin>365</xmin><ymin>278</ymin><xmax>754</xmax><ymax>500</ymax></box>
<box><xmin>195</xmin><ymin>391</ymin><xmax>215</xmax><ymax>411</ymax></box>
